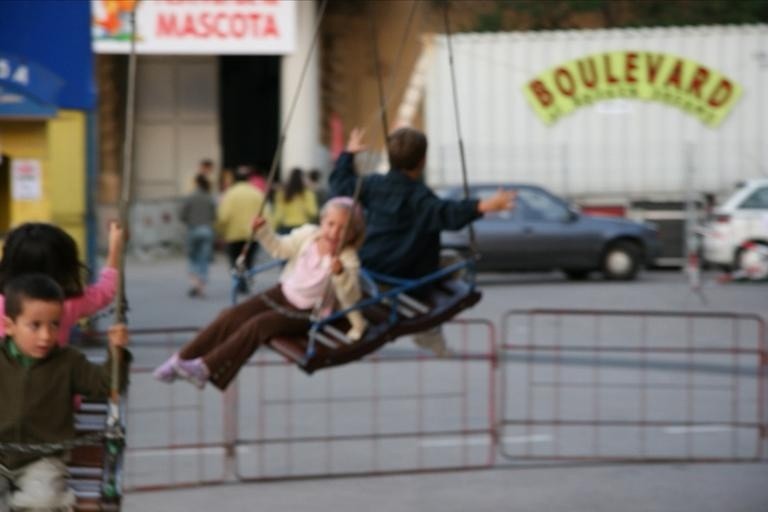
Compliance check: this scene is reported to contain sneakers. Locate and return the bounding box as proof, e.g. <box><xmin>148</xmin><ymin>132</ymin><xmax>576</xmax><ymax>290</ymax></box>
<box><xmin>171</xmin><ymin>356</ymin><xmax>209</xmax><ymax>390</ymax></box>
<box><xmin>414</xmin><ymin>331</ymin><xmax>455</xmax><ymax>358</ymax></box>
<box><xmin>151</xmin><ymin>352</ymin><xmax>182</xmax><ymax>384</ymax></box>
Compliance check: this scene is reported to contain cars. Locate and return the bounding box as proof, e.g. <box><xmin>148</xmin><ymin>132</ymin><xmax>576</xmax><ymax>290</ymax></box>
<box><xmin>429</xmin><ymin>178</ymin><xmax>662</xmax><ymax>281</ymax></box>
<box><xmin>703</xmin><ymin>174</ymin><xmax>768</xmax><ymax>283</ymax></box>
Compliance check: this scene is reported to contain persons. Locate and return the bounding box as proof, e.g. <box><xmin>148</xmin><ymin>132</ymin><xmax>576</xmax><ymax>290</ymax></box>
<box><xmin>326</xmin><ymin>122</ymin><xmax>518</xmax><ymax>357</ymax></box>
<box><xmin>176</xmin><ymin>154</ymin><xmax>327</xmax><ymax>301</ymax></box>
<box><xmin>152</xmin><ymin>194</ymin><xmax>367</xmax><ymax>390</ymax></box>
<box><xmin>0</xmin><ymin>271</ymin><xmax>135</xmax><ymax>511</ymax></box>
<box><xmin>0</xmin><ymin>219</ymin><xmax>125</xmax><ymax>413</ymax></box>
